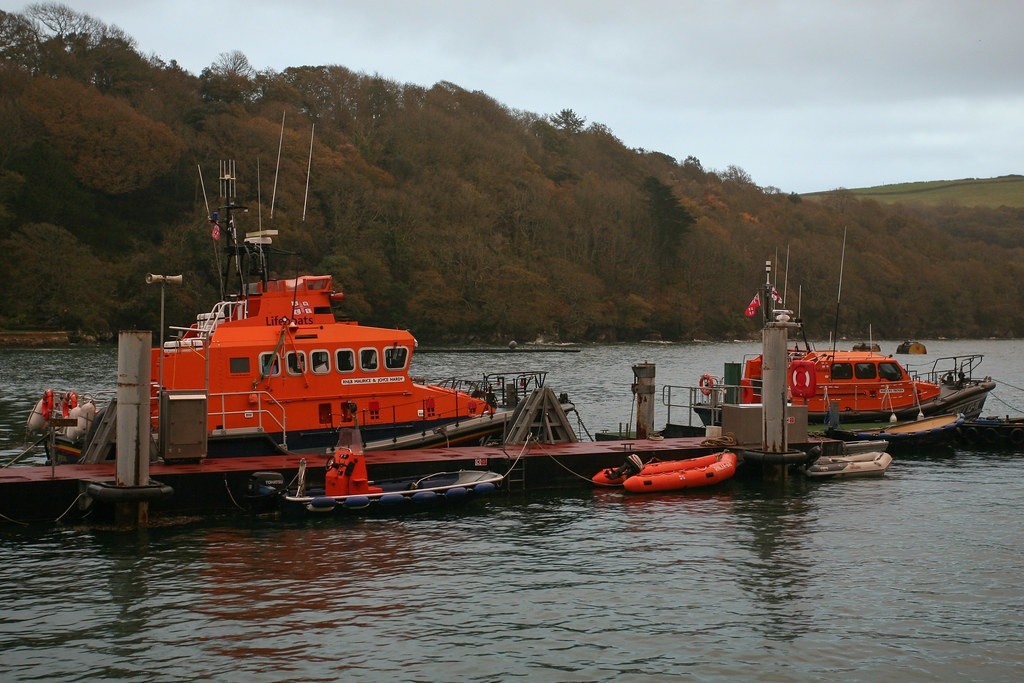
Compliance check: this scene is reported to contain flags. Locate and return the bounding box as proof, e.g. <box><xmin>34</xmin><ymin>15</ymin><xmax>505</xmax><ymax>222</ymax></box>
<box><xmin>772</xmin><ymin>287</ymin><xmax>783</xmax><ymax>304</ymax></box>
<box><xmin>744</xmin><ymin>292</ymin><xmax>761</xmax><ymax>318</ymax></box>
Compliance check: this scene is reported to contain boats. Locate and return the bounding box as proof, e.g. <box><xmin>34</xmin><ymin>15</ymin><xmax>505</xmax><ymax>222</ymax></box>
<box><xmin>22</xmin><ymin>110</ymin><xmax>581</xmax><ymax>521</ymax></box>
<box><xmin>240</xmin><ymin>399</ymin><xmax>507</xmax><ymax>526</ymax></box>
<box><xmin>797</xmin><ymin>451</ymin><xmax>893</xmax><ymax>480</ymax></box>
<box><xmin>824</xmin><ymin>412</ymin><xmax>965</xmax><ymax>453</ymax></box>
<box><xmin>840</xmin><ymin>439</ymin><xmax>889</xmax><ymax>455</ymax></box>
<box><xmin>692</xmin><ymin>223</ymin><xmax>995</xmax><ymax>426</ymax></box>
<box><xmin>591</xmin><ymin>449</ymin><xmax>737</xmax><ymax>494</ymax></box>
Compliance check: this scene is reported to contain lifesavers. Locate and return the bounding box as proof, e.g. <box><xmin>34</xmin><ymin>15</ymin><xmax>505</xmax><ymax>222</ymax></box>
<box><xmin>1009</xmin><ymin>428</ymin><xmax>1024</xmax><ymax>445</ymax></box>
<box><xmin>698</xmin><ymin>374</ymin><xmax>714</xmax><ymax>396</ymax></box>
<box><xmin>952</xmin><ymin>427</ymin><xmax>963</xmax><ymax>444</ymax></box>
<box><xmin>966</xmin><ymin>427</ymin><xmax>980</xmax><ymax>445</ymax></box>
<box><xmin>40</xmin><ymin>388</ymin><xmax>53</xmax><ymax>421</ymax></box>
<box><xmin>62</xmin><ymin>391</ymin><xmax>78</xmax><ymax>419</ymax></box>
<box><xmin>982</xmin><ymin>428</ymin><xmax>1000</xmax><ymax>444</ymax></box>
